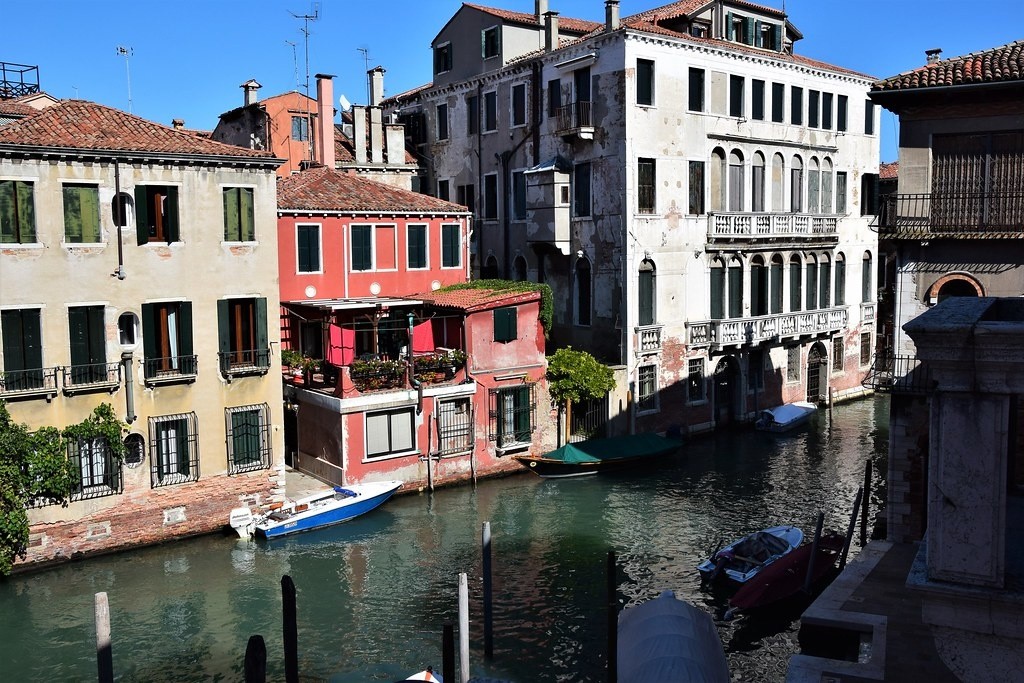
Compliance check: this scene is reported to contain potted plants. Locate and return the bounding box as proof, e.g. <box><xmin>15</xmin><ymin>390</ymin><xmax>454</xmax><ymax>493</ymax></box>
<box><xmin>280</xmin><ymin>349</ymin><xmax>320</xmax><ymax>378</ymax></box>
<box><xmin>348</xmin><ymin>350</ymin><xmax>468</xmax><ymax>393</ymax></box>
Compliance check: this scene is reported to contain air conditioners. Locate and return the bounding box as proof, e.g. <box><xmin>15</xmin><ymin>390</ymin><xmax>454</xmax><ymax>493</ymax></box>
<box><xmin>436</xmin><ymin>347</ymin><xmax>456</xmax><ymax>358</ymax></box>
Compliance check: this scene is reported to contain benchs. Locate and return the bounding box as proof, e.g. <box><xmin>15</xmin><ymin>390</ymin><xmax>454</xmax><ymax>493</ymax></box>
<box><xmin>270</xmin><ymin>512</ymin><xmax>290</xmax><ymax>521</ymax></box>
<box><xmin>732</xmin><ymin>555</ymin><xmax>765</xmax><ymax>566</ymax></box>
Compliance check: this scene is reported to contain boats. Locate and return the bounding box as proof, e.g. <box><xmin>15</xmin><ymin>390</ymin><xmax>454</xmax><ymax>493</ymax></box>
<box><xmin>511</xmin><ymin>430</ymin><xmax>687</xmax><ymax>479</ymax></box>
<box><xmin>229</xmin><ymin>479</ymin><xmax>406</xmax><ymax>543</ymax></box>
<box><xmin>754</xmin><ymin>400</ymin><xmax>818</xmax><ymax>434</ymax></box>
<box><xmin>618</xmin><ymin>589</ymin><xmax>732</xmax><ymax>683</ymax></box>
<box><xmin>404</xmin><ymin>664</ymin><xmax>444</xmax><ymax>683</ymax></box>
<box><xmin>696</xmin><ymin>524</ymin><xmax>803</xmax><ymax>600</ymax></box>
